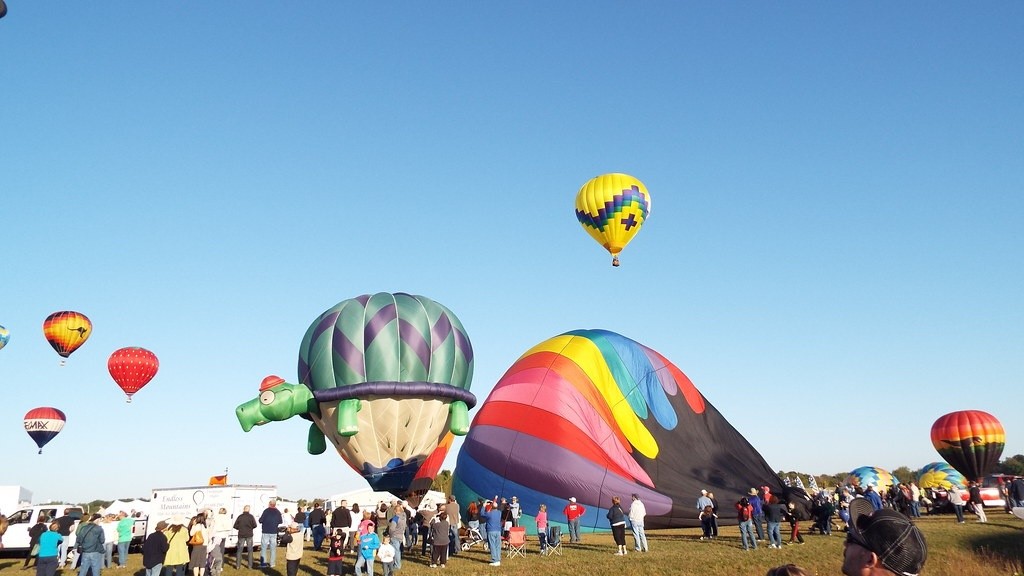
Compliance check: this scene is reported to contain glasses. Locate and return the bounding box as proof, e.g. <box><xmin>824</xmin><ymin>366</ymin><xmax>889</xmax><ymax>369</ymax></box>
<box><xmin>847</xmin><ymin>530</ymin><xmax>873</xmax><ymax>552</ymax></box>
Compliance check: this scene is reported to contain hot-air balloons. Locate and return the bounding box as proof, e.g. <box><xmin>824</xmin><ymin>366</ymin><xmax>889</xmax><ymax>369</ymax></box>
<box><xmin>43</xmin><ymin>311</ymin><xmax>92</xmax><ymax>366</ymax></box>
<box><xmin>107</xmin><ymin>346</ymin><xmax>159</xmax><ymax>403</ymax></box>
<box><xmin>574</xmin><ymin>173</ymin><xmax>651</xmax><ymax>267</ymax></box>
<box><xmin>844</xmin><ymin>467</ymin><xmax>901</xmax><ymax>498</ymax></box>
<box><xmin>24</xmin><ymin>407</ymin><xmax>67</xmax><ymax>454</ymax></box>
<box><xmin>930</xmin><ymin>410</ymin><xmax>1005</xmax><ymax>489</ymax></box>
<box><xmin>448</xmin><ymin>329</ymin><xmax>816</xmax><ymax>536</ymax></box>
<box><xmin>234</xmin><ymin>291</ymin><xmax>474</xmax><ymax>511</ymax></box>
<box><xmin>0</xmin><ymin>325</ymin><xmax>11</xmax><ymax>349</ymax></box>
<box><xmin>916</xmin><ymin>461</ymin><xmax>968</xmax><ymax>497</ymax></box>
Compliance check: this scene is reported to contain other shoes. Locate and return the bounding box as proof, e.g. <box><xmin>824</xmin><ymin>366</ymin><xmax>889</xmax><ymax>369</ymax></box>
<box><xmin>808</xmin><ymin>526</ymin><xmax>815</xmax><ymax>531</ymax></box>
<box><xmin>489</xmin><ymin>561</ymin><xmax>500</xmax><ymax>566</ymax></box>
<box><xmin>787</xmin><ymin>542</ymin><xmax>794</xmax><ymax>546</ymax></box>
<box><xmin>838</xmin><ymin>526</ymin><xmax>840</xmax><ymax>530</ymax></box>
<box><xmin>622</xmin><ymin>551</ymin><xmax>627</xmax><ymax>554</ymax></box>
<box><xmin>777</xmin><ymin>546</ymin><xmax>781</xmax><ymax>549</ymax></box>
<box><xmin>769</xmin><ymin>544</ymin><xmax>777</xmax><ymax>548</ymax></box>
<box><xmin>441</xmin><ymin>564</ymin><xmax>445</xmax><ymax>568</ymax></box>
<box><xmin>614</xmin><ymin>551</ymin><xmax>623</xmax><ymax>556</ymax></box>
<box><xmin>798</xmin><ymin>541</ymin><xmax>805</xmax><ymax>545</ymax></box>
<box><xmin>429</xmin><ymin>564</ymin><xmax>437</xmax><ymax>568</ymax></box>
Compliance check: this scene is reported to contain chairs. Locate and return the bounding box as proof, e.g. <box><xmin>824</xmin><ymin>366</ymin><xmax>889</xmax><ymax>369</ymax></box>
<box><xmin>544</xmin><ymin>526</ymin><xmax>562</xmax><ymax>557</ymax></box>
<box><xmin>501</xmin><ymin>526</ymin><xmax>526</xmax><ymax>559</ymax></box>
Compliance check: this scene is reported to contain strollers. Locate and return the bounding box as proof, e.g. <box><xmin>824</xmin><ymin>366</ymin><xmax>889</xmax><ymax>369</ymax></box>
<box><xmin>461</xmin><ymin>528</ymin><xmax>489</xmax><ymax>551</ymax></box>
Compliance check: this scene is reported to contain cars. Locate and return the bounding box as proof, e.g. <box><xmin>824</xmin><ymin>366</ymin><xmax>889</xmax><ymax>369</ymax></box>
<box><xmin>1</xmin><ymin>505</ymin><xmax>85</xmax><ymax>564</ymax></box>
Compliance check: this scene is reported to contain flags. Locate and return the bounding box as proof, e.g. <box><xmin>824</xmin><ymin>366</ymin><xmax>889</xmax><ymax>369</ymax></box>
<box><xmin>209</xmin><ymin>475</ymin><xmax>227</xmax><ymax>486</ymax></box>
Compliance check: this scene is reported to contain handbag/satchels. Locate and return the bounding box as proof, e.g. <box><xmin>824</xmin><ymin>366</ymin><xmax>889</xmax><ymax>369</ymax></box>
<box><xmin>30</xmin><ymin>544</ymin><xmax>39</xmax><ymax>556</ymax></box>
<box><xmin>189</xmin><ymin>530</ymin><xmax>204</xmax><ymax>545</ymax></box>
<box><xmin>426</xmin><ymin>538</ymin><xmax>434</xmax><ymax>545</ymax></box>
<box><xmin>77</xmin><ymin>545</ymin><xmax>83</xmax><ymax>554</ymax></box>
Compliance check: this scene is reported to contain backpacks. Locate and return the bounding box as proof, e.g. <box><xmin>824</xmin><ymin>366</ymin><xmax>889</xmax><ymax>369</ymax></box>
<box><xmin>740</xmin><ymin>507</ymin><xmax>749</xmax><ymax>521</ymax></box>
<box><xmin>828</xmin><ymin>504</ymin><xmax>836</xmax><ymax>516</ymax></box>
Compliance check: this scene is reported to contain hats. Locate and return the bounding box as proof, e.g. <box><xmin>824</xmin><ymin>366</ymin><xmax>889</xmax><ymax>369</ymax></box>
<box><xmin>868</xmin><ymin>483</ymin><xmax>875</xmax><ymax>486</ymax></box>
<box><xmin>849</xmin><ymin>497</ymin><xmax>928</xmax><ymax>576</ymax></box>
<box><xmin>155</xmin><ymin>521</ymin><xmax>169</xmax><ymax>530</ymax></box>
<box><xmin>701</xmin><ymin>490</ymin><xmax>707</xmax><ymax>494</ymax></box>
<box><xmin>748</xmin><ymin>488</ymin><xmax>759</xmax><ymax>495</ymax></box>
<box><xmin>288</xmin><ymin>522</ymin><xmax>299</xmax><ymax>528</ymax></box>
<box><xmin>569</xmin><ymin>497</ymin><xmax>576</xmax><ymax>502</ymax></box>
<box><xmin>761</xmin><ymin>486</ymin><xmax>769</xmax><ymax>492</ymax></box>
<box><xmin>165</xmin><ymin>515</ymin><xmax>185</xmax><ymax>524</ymax></box>
<box><xmin>89</xmin><ymin>514</ymin><xmax>101</xmax><ymax>522</ymax></box>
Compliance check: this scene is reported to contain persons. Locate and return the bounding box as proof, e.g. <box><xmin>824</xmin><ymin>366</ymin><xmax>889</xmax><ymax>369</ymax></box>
<box><xmin>766</xmin><ymin>564</ymin><xmax>812</xmax><ymax>576</ymax></box>
<box><xmin>735</xmin><ymin>485</ymin><xmax>805</xmax><ymax>551</ymax></box>
<box><xmin>233</xmin><ymin>505</ymin><xmax>257</xmax><ymax>570</ymax></box>
<box><xmin>0</xmin><ymin>513</ymin><xmax>9</xmax><ymax>549</ymax></box>
<box><xmin>696</xmin><ymin>489</ymin><xmax>718</xmax><ymax>540</ymax></box>
<box><xmin>467</xmin><ymin>495</ymin><xmax>523</xmax><ymax>567</ymax></box>
<box><xmin>19</xmin><ymin>508</ymin><xmax>231</xmax><ymax>576</ymax></box>
<box><xmin>535</xmin><ymin>504</ymin><xmax>547</xmax><ymax>549</ymax></box>
<box><xmin>628</xmin><ymin>493</ymin><xmax>649</xmax><ymax>552</ymax></box>
<box><xmin>807</xmin><ymin>476</ymin><xmax>1024</xmax><ymax>537</ymax></box>
<box><xmin>259</xmin><ymin>500</ymin><xmax>283</xmax><ymax>568</ymax></box>
<box><xmin>606</xmin><ymin>496</ymin><xmax>627</xmax><ymax>555</ymax></box>
<box><xmin>563</xmin><ymin>497</ymin><xmax>586</xmax><ymax>543</ymax></box>
<box><xmin>841</xmin><ymin>498</ymin><xmax>927</xmax><ymax>576</ymax></box>
<box><xmin>282</xmin><ymin>495</ymin><xmax>460</xmax><ymax>576</ymax></box>
